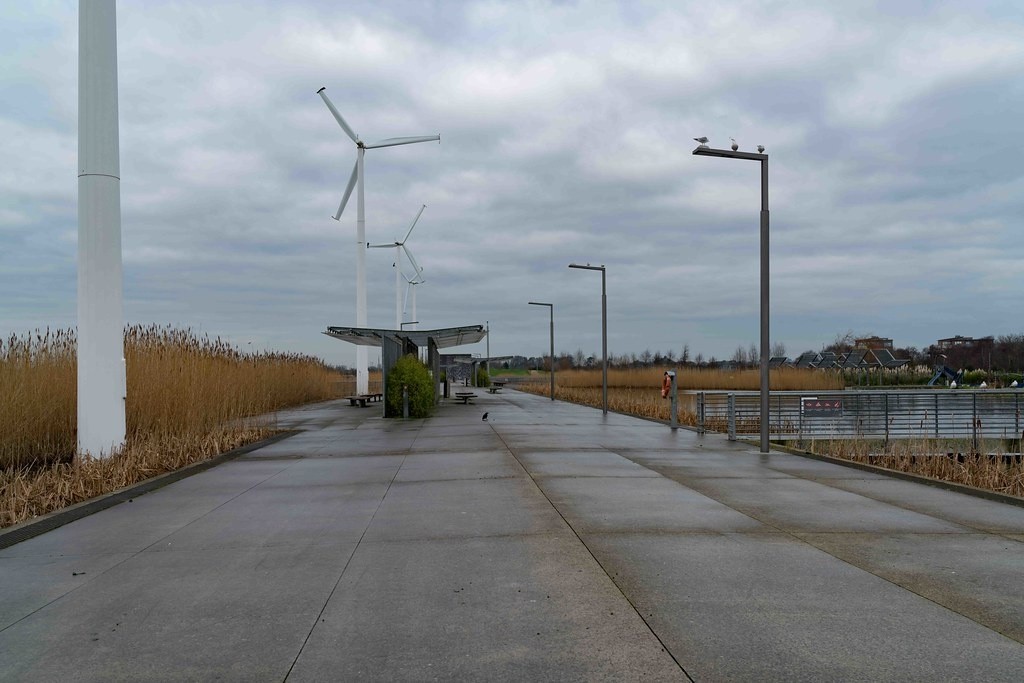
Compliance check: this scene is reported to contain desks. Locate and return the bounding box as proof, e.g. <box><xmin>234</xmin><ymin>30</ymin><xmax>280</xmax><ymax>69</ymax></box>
<box><xmin>487</xmin><ymin>387</ymin><xmax>503</xmax><ymax>395</ymax></box>
<box><xmin>493</xmin><ymin>382</ymin><xmax>506</xmax><ymax>387</ymax></box>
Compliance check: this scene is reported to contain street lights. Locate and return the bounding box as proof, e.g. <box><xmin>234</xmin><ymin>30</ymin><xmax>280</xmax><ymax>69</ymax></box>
<box><xmin>569</xmin><ymin>263</ymin><xmax>607</xmax><ymax>415</ymax></box>
<box><xmin>693</xmin><ymin>138</ymin><xmax>770</xmax><ymax>453</ymax></box>
<box><xmin>528</xmin><ymin>302</ymin><xmax>555</xmax><ymax>400</ymax></box>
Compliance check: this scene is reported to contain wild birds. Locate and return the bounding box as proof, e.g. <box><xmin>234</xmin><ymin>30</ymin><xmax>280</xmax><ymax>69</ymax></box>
<box><xmin>949</xmin><ymin>380</ymin><xmax>957</xmax><ymax>389</ymax></box>
<box><xmin>1009</xmin><ymin>380</ymin><xmax>1018</xmax><ymax>388</ymax></box>
<box><xmin>482</xmin><ymin>412</ymin><xmax>489</xmax><ymax>421</ymax></box>
<box><xmin>979</xmin><ymin>380</ymin><xmax>988</xmax><ymax>389</ymax></box>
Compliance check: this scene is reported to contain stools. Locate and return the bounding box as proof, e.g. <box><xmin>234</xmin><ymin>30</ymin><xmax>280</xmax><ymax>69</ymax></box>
<box><xmin>455</xmin><ymin>392</ymin><xmax>478</xmax><ymax>405</ymax></box>
<box><xmin>345</xmin><ymin>394</ymin><xmax>382</xmax><ymax>408</ymax></box>
